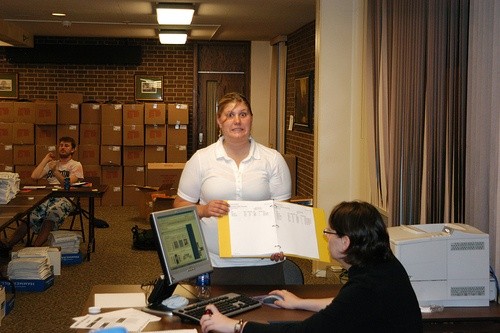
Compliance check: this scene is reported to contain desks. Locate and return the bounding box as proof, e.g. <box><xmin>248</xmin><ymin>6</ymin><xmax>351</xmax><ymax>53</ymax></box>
<box><xmin>0</xmin><ymin>183</ymin><xmax>109</xmax><ymax>261</ymax></box>
<box><xmin>70</xmin><ymin>284</ymin><xmax>500</xmax><ymax>333</ymax></box>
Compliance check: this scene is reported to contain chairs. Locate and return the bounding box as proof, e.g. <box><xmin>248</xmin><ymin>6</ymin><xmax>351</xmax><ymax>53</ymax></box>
<box><xmin>29</xmin><ymin>177</ymin><xmax>97</xmax><ymax>242</ymax></box>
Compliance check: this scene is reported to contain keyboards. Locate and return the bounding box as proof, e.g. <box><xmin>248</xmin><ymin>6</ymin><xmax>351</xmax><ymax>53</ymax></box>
<box><xmin>172</xmin><ymin>293</ymin><xmax>260</xmax><ymax>324</ymax></box>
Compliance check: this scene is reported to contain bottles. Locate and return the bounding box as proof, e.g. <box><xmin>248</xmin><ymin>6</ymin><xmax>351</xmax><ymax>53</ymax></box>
<box><xmin>64</xmin><ymin>177</ymin><xmax>70</xmax><ymax>189</ymax></box>
<box><xmin>196</xmin><ymin>272</ymin><xmax>210</xmax><ymax>300</ymax></box>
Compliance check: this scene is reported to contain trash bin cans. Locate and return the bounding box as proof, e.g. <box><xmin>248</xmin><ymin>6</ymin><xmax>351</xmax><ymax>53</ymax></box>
<box><xmin>153</xmin><ymin>198</ymin><xmax>175</xmax><ymax>212</ymax></box>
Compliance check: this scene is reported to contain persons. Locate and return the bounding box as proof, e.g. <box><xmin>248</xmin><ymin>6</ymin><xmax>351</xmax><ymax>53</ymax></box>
<box><xmin>199</xmin><ymin>202</ymin><xmax>423</xmax><ymax>333</ymax></box>
<box><xmin>234</xmin><ymin>319</ymin><xmax>247</xmax><ymax>333</ymax></box>
<box><xmin>173</xmin><ymin>91</ymin><xmax>292</xmax><ymax>286</ymax></box>
<box><xmin>0</xmin><ymin>136</ymin><xmax>84</xmax><ymax>246</ymax></box>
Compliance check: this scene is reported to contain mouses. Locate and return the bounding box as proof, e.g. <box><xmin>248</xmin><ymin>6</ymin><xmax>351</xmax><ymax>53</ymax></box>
<box><xmin>262</xmin><ymin>295</ymin><xmax>285</xmax><ymax>308</ymax></box>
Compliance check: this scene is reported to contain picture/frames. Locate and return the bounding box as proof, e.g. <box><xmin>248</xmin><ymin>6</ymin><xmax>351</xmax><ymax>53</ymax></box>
<box><xmin>0</xmin><ymin>72</ymin><xmax>19</xmax><ymax>99</ymax></box>
<box><xmin>293</xmin><ymin>69</ymin><xmax>314</xmax><ymax>133</ymax></box>
<box><xmin>133</xmin><ymin>74</ymin><xmax>164</xmax><ymax>102</ymax></box>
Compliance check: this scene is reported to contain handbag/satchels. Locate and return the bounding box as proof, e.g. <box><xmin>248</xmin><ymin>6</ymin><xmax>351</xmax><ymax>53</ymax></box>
<box><xmin>131</xmin><ymin>224</ymin><xmax>157</xmax><ymax>250</ymax></box>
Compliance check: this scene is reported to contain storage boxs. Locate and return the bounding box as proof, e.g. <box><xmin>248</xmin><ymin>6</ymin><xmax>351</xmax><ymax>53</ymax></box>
<box><xmin>0</xmin><ymin>276</ymin><xmax>54</xmax><ymax>321</ymax></box>
<box><xmin>283</xmin><ymin>153</ymin><xmax>296</xmax><ymax>196</ymax></box>
<box><xmin>0</xmin><ymin>92</ymin><xmax>188</xmax><ymax>221</ymax></box>
<box><xmin>60</xmin><ymin>252</ymin><xmax>83</xmax><ymax>265</ymax></box>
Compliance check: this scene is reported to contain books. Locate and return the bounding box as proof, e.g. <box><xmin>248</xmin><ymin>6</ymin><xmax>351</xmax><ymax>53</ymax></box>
<box><xmin>7</xmin><ymin>246</ymin><xmax>61</xmax><ymax>280</ymax></box>
<box><xmin>218</xmin><ymin>200</ymin><xmax>331</xmax><ymax>264</ymax></box>
<box><xmin>49</xmin><ymin>231</ymin><xmax>80</xmax><ymax>254</ymax></box>
<box><xmin>0</xmin><ymin>172</ymin><xmax>20</xmax><ymax>204</ymax></box>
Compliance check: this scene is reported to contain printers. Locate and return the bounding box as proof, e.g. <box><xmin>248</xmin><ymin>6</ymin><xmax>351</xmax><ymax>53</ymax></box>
<box><xmin>388</xmin><ymin>222</ymin><xmax>490</xmax><ymax>307</ymax></box>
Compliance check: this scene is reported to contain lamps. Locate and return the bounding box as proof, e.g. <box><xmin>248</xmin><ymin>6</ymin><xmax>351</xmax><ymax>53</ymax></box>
<box><xmin>156</xmin><ymin>3</ymin><xmax>195</xmax><ymax>25</ymax></box>
<box><xmin>158</xmin><ymin>31</ymin><xmax>188</xmax><ymax>45</ymax></box>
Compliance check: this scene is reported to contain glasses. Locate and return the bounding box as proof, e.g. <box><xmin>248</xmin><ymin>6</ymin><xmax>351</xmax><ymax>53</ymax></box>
<box><xmin>323</xmin><ymin>228</ymin><xmax>350</xmax><ymax>241</ymax></box>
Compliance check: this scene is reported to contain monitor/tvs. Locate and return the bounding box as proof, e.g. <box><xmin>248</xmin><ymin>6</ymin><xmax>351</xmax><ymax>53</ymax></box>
<box><xmin>151</xmin><ymin>205</ymin><xmax>214</xmax><ymax>287</ymax></box>
